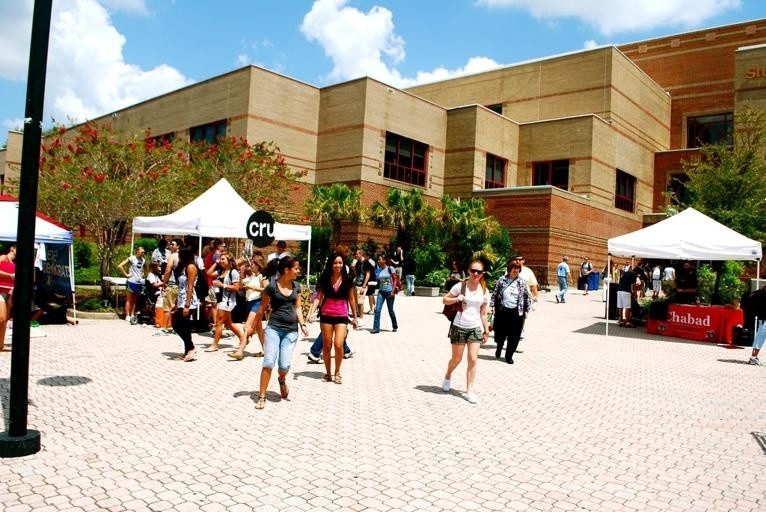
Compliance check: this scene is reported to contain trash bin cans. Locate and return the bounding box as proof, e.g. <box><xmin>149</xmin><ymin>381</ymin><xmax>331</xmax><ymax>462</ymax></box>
<box><xmin>594</xmin><ymin>272</ymin><xmax>599</xmax><ymax>289</ymax></box>
<box><xmin>588</xmin><ymin>272</ymin><xmax>594</xmax><ymax>290</ymax></box>
<box><xmin>578</xmin><ymin>277</ymin><xmax>584</xmax><ymax>290</ymax></box>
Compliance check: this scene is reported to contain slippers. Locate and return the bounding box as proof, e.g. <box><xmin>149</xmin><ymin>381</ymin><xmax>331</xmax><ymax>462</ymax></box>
<box><xmin>256</xmin><ymin>397</ymin><xmax>266</xmax><ymax>409</ymax></box>
<box><xmin>278</xmin><ymin>377</ymin><xmax>289</xmax><ymax>398</ymax></box>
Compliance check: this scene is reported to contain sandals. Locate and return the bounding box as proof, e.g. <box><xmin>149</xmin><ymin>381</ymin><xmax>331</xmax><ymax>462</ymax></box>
<box><xmin>322</xmin><ymin>374</ymin><xmax>331</xmax><ymax>382</ymax></box>
<box><xmin>335</xmin><ymin>372</ymin><xmax>342</xmax><ymax>384</ymax></box>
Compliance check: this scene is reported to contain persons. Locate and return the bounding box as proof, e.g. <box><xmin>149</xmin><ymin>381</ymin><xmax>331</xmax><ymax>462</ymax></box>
<box><xmin>402</xmin><ymin>257</ymin><xmax>417</xmax><ymax>296</ymax></box>
<box><xmin>442</xmin><ymin>260</ymin><xmax>465</xmax><ymax>313</ymax></box>
<box><xmin>555</xmin><ymin>256</ymin><xmax>573</xmax><ymax>303</ymax></box>
<box><xmin>0</xmin><ymin>244</ymin><xmax>17</xmax><ymax>351</ymax></box>
<box><xmin>115</xmin><ymin>241</ymin><xmax>292</xmax><ymax>362</ymax></box>
<box><xmin>443</xmin><ymin>260</ymin><xmax>490</xmax><ymax>404</ymax></box>
<box><xmin>601</xmin><ymin>258</ymin><xmax>701</xmax><ymax>329</ymax></box>
<box><xmin>35</xmin><ymin>273</ymin><xmax>65</xmax><ymax>318</ymax></box>
<box><xmin>748</xmin><ymin>285</ymin><xmax>766</xmax><ymax>365</ymax></box>
<box><xmin>390</xmin><ymin>245</ymin><xmax>403</xmax><ymax>280</ymax></box>
<box><xmin>309</xmin><ymin>241</ymin><xmax>399</xmax><ymax>384</ymax></box>
<box><xmin>505</xmin><ymin>254</ymin><xmax>538</xmax><ymax>352</ymax></box>
<box><xmin>580</xmin><ymin>256</ymin><xmax>593</xmax><ymax>296</ymax></box>
<box><xmin>490</xmin><ymin>262</ymin><xmax>532</xmax><ymax>363</ymax></box>
<box><xmin>246</xmin><ymin>256</ymin><xmax>308</xmax><ymax>409</ymax></box>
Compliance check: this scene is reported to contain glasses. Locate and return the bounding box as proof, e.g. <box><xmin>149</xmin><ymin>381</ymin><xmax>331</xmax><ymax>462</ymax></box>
<box><xmin>470</xmin><ymin>269</ymin><xmax>483</xmax><ymax>274</ymax></box>
<box><xmin>515</xmin><ymin>259</ymin><xmax>523</xmax><ymax>261</ymax></box>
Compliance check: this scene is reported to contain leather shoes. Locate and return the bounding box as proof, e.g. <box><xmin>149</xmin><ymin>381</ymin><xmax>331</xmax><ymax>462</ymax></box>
<box><xmin>505</xmin><ymin>356</ymin><xmax>513</xmax><ymax>364</ymax></box>
<box><xmin>496</xmin><ymin>349</ymin><xmax>501</xmax><ymax>358</ymax></box>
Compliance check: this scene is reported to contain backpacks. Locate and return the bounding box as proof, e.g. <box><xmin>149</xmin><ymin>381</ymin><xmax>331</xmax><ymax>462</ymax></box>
<box><xmin>195</xmin><ymin>270</ymin><xmax>210</xmax><ymax>299</ymax></box>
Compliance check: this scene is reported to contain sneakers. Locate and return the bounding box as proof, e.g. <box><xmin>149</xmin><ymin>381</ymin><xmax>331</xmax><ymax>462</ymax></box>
<box><xmin>370</xmin><ymin>330</ymin><xmax>379</xmax><ymax>334</ymax></box>
<box><xmin>392</xmin><ymin>328</ymin><xmax>398</xmax><ymax>331</ymax></box>
<box><xmin>204</xmin><ymin>346</ymin><xmax>218</xmax><ymax>352</ymax></box>
<box><xmin>561</xmin><ymin>299</ymin><xmax>565</xmax><ymax>303</ymax></box>
<box><xmin>308</xmin><ymin>353</ymin><xmax>324</xmax><ymax>364</ymax></box>
<box><xmin>442</xmin><ymin>377</ymin><xmax>451</xmax><ymax>392</ymax></box>
<box><xmin>227</xmin><ymin>353</ymin><xmax>245</xmax><ymax>360</ymax></box>
<box><xmin>344</xmin><ymin>353</ymin><xmax>354</xmax><ymax>359</ymax></box>
<box><xmin>555</xmin><ymin>294</ymin><xmax>560</xmax><ymax>303</ymax></box>
<box><xmin>254</xmin><ymin>352</ymin><xmax>265</xmax><ymax>357</ymax></box>
<box><xmin>619</xmin><ymin>321</ymin><xmax>626</xmax><ymax>327</ymax></box>
<box><xmin>156</xmin><ymin>328</ymin><xmax>168</xmax><ymax>336</ymax></box>
<box><xmin>125</xmin><ymin>315</ymin><xmax>131</xmax><ymax>321</ymax></box>
<box><xmin>626</xmin><ymin>322</ymin><xmax>636</xmax><ymax>328</ymax></box>
<box><xmin>749</xmin><ymin>357</ymin><xmax>761</xmax><ymax>365</ymax></box>
<box><xmin>184</xmin><ymin>349</ymin><xmax>198</xmax><ymax>362</ymax></box>
<box><xmin>464</xmin><ymin>393</ymin><xmax>478</xmax><ymax>404</ymax></box>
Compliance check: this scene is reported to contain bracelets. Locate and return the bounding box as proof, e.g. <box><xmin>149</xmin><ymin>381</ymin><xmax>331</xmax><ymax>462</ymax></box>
<box><xmin>300</xmin><ymin>323</ymin><xmax>306</xmax><ymax>329</ymax></box>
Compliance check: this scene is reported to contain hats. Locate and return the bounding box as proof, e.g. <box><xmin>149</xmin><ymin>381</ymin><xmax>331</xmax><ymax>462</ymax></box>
<box><xmin>563</xmin><ymin>255</ymin><xmax>569</xmax><ymax>260</ymax></box>
<box><xmin>254</xmin><ymin>255</ymin><xmax>267</xmax><ymax>271</ymax></box>
<box><xmin>276</xmin><ymin>241</ymin><xmax>287</xmax><ymax>248</ymax></box>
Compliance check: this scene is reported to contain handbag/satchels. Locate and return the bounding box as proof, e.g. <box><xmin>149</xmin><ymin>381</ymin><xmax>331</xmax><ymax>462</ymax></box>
<box><xmin>442</xmin><ymin>281</ymin><xmax>466</xmax><ymax>321</ymax></box>
<box><xmin>446</xmin><ymin>278</ymin><xmax>460</xmax><ymax>291</ymax></box>
<box><xmin>391</xmin><ymin>273</ymin><xmax>403</xmax><ymax>294</ymax></box>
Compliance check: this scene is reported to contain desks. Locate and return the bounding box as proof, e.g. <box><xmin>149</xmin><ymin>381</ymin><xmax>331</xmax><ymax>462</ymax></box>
<box><xmin>647</xmin><ymin>305</ymin><xmax>744</xmax><ymax>344</ymax></box>
<box><xmin>103</xmin><ymin>276</ymin><xmax>127</xmax><ymax>312</ymax></box>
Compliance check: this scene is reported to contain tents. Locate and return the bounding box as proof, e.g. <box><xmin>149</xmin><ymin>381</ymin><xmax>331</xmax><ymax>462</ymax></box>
<box><xmin>130</xmin><ymin>179</ymin><xmax>311</xmax><ymax>285</ymax></box>
